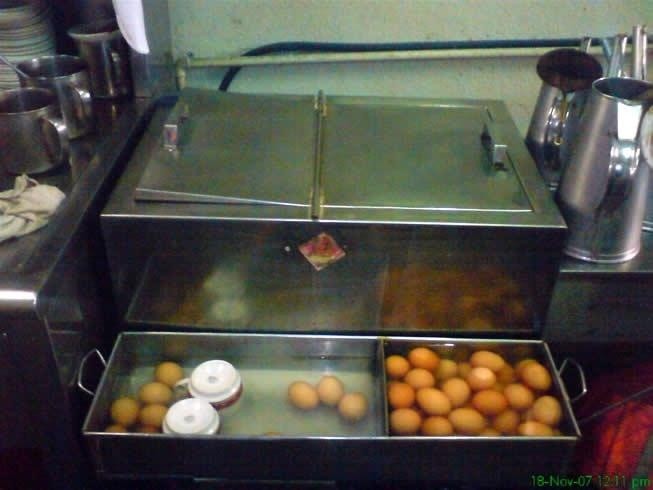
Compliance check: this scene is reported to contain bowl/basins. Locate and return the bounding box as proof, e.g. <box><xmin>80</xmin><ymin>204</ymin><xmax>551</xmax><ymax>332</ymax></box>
<box><xmin>0</xmin><ymin>0</ymin><xmax>42</xmax><ymax>29</ymax></box>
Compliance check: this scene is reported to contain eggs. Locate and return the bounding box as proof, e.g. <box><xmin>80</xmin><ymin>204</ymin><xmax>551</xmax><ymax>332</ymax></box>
<box><xmin>386</xmin><ymin>347</ymin><xmax>564</xmax><ymax>436</ymax></box>
<box><xmin>103</xmin><ymin>361</ymin><xmax>183</xmax><ymax>432</ymax></box>
<box><xmin>288</xmin><ymin>381</ymin><xmax>320</xmax><ymax>410</ymax></box>
<box><xmin>381</xmin><ymin>263</ymin><xmax>528</xmax><ymax>329</ymax></box>
<box><xmin>261</xmin><ymin>430</ymin><xmax>281</xmax><ymax>435</ymax></box>
<box><xmin>150</xmin><ymin>268</ymin><xmax>234</xmax><ymax>359</ymax></box>
<box><xmin>338</xmin><ymin>391</ymin><xmax>369</xmax><ymax>424</ymax></box>
<box><xmin>317</xmin><ymin>375</ymin><xmax>345</xmax><ymax>408</ymax></box>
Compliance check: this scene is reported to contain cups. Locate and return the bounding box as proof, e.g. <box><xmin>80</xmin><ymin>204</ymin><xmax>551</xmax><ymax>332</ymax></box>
<box><xmin>163</xmin><ymin>359</ymin><xmax>244</xmax><ymax>437</ymax></box>
<box><xmin>524</xmin><ymin>48</ymin><xmax>653</xmax><ymax>264</ymax></box>
<box><xmin>0</xmin><ymin>19</ymin><xmax>131</xmax><ymax>175</ymax></box>
